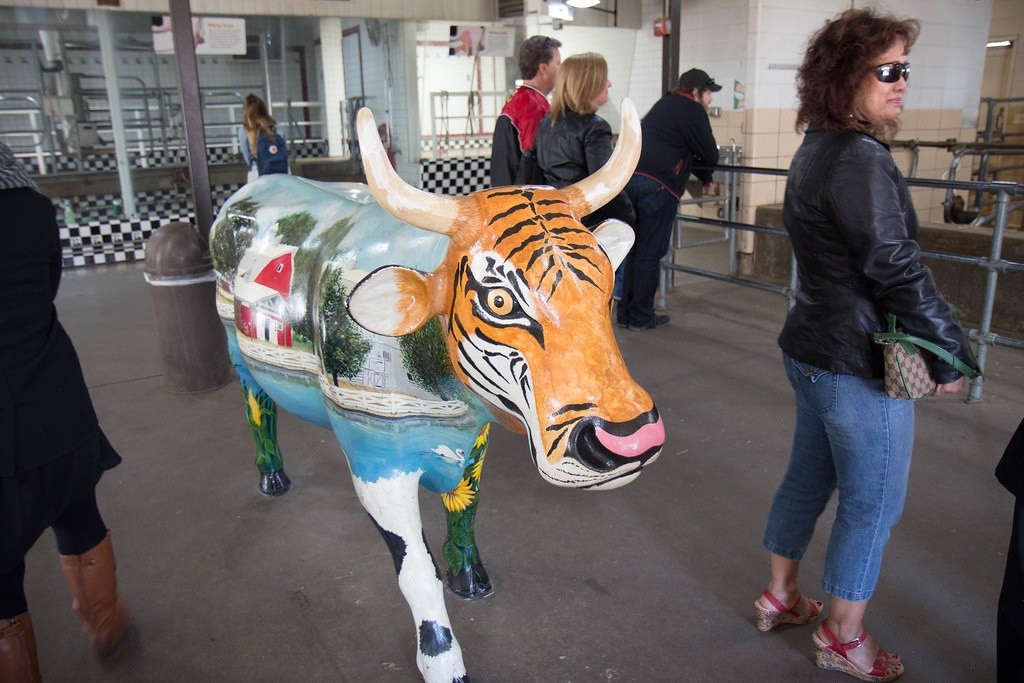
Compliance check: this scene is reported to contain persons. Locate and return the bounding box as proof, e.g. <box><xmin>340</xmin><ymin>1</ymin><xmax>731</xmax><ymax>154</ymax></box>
<box><xmin>238</xmin><ymin>94</ymin><xmax>292</xmax><ymax>184</ymax></box>
<box><xmin>0</xmin><ymin>141</ymin><xmax>129</xmax><ymax>683</ymax></box>
<box><xmin>490</xmin><ymin>35</ymin><xmax>636</xmax><ymax>301</ymax></box>
<box><xmin>617</xmin><ymin>68</ymin><xmax>722</xmax><ymax>332</ymax></box>
<box><xmin>753</xmin><ymin>7</ymin><xmax>978</xmax><ymax>683</ymax></box>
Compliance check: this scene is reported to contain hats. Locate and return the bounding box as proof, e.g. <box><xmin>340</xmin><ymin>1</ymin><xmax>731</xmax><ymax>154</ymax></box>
<box><xmin>679</xmin><ymin>68</ymin><xmax>722</xmax><ymax>92</ymax></box>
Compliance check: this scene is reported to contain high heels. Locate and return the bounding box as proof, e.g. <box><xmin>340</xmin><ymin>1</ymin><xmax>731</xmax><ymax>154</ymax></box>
<box><xmin>755</xmin><ymin>589</ymin><xmax>819</xmax><ymax>632</ymax></box>
<box><xmin>812</xmin><ymin>619</ymin><xmax>904</xmax><ymax>681</ymax></box>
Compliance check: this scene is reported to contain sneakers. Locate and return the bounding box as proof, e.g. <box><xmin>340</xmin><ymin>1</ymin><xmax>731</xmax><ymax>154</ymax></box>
<box><xmin>617</xmin><ymin>312</ymin><xmax>670</xmax><ymax>330</ymax></box>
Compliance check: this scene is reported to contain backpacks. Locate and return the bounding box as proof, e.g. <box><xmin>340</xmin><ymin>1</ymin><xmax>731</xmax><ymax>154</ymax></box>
<box><xmin>248</xmin><ymin>126</ymin><xmax>289</xmax><ymax>175</ymax></box>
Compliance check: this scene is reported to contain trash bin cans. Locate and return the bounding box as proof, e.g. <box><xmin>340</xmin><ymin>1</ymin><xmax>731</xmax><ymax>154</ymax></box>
<box><xmin>143</xmin><ymin>221</ymin><xmax>233</xmax><ymax>395</ymax></box>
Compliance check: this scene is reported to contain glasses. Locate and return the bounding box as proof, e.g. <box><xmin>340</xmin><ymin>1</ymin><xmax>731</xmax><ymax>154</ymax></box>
<box><xmin>867</xmin><ymin>62</ymin><xmax>911</xmax><ymax>83</ymax></box>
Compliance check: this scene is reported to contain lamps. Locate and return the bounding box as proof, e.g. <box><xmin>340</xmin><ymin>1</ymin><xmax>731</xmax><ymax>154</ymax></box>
<box><xmin>38</xmin><ymin>26</ymin><xmax>68</xmax><ymax>76</ymax></box>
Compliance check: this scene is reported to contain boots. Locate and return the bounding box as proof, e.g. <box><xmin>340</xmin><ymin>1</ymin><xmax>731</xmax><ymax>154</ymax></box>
<box><xmin>0</xmin><ymin>610</ymin><xmax>42</xmax><ymax>683</ymax></box>
<box><xmin>60</xmin><ymin>529</ymin><xmax>133</xmax><ymax>662</ymax></box>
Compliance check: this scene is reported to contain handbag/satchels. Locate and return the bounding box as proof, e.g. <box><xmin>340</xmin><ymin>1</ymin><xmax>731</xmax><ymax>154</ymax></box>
<box><xmin>874</xmin><ymin>300</ymin><xmax>980</xmax><ymax>400</ymax></box>
